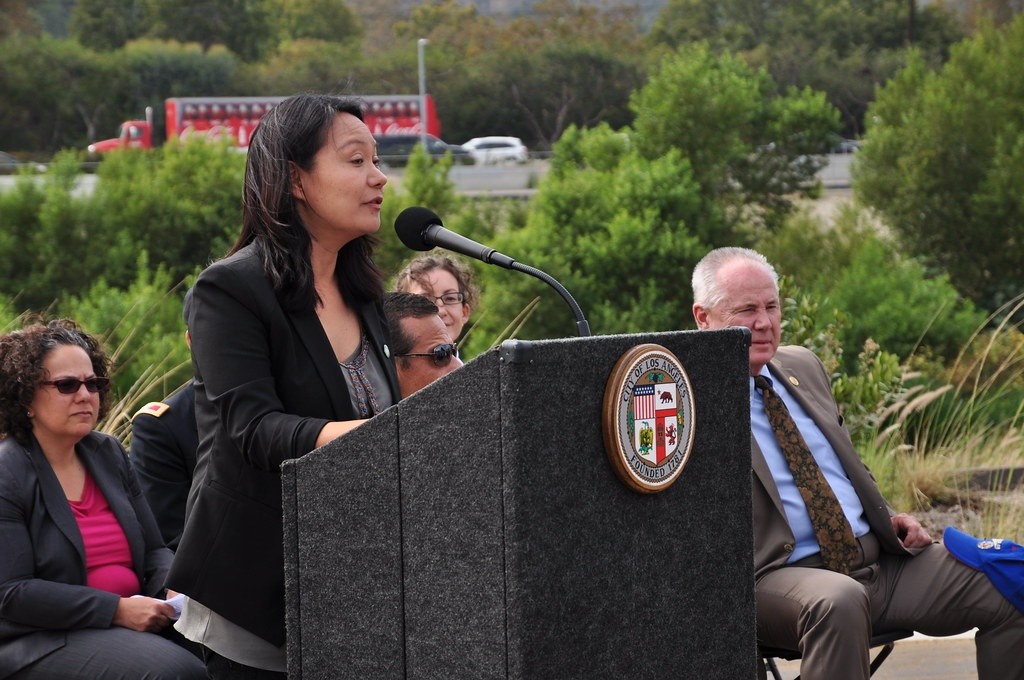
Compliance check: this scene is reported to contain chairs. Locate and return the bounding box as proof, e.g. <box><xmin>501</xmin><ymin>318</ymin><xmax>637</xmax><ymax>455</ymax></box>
<box><xmin>758</xmin><ymin>629</ymin><xmax>917</xmax><ymax>680</ymax></box>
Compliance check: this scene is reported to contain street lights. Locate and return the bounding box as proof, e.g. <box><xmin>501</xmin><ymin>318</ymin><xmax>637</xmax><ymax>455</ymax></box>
<box><xmin>416</xmin><ymin>38</ymin><xmax>428</xmax><ymax>152</ymax></box>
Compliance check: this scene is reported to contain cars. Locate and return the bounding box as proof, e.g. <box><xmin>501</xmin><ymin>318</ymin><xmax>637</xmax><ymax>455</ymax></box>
<box><xmin>370</xmin><ymin>135</ymin><xmax>475</xmax><ymax>170</ymax></box>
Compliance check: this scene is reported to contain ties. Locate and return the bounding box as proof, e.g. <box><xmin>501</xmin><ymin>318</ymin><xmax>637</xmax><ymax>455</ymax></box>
<box><xmin>754</xmin><ymin>374</ymin><xmax>857</xmax><ymax>576</ymax></box>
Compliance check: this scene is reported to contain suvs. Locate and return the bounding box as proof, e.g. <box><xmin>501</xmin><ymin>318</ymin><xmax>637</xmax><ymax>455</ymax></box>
<box><xmin>462</xmin><ymin>136</ymin><xmax>528</xmax><ymax>166</ymax></box>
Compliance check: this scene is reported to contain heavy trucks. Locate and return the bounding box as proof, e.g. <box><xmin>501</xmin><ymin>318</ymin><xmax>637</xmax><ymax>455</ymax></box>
<box><xmin>88</xmin><ymin>95</ymin><xmax>443</xmax><ymax>159</ymax></box>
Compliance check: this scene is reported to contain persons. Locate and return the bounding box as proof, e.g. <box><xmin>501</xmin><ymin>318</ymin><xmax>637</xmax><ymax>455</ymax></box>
<box><xmin>692</xmin><ymin>248</ymin><xmax>1024</xmax><ymax>680</ymax></box>
<box><xmin>0</xmin><ymin>315</ymin><xmax>239</xmax><ymax>680</ymax></box>
<box><xmin>163</xmin><ymin>94</ymin><xmax>403</xmax><ymax>680</ymax></box>
<box><xmin>129</xmin><ymin>287</ymin><xmax>201</xmax><ymax>557</ymax></box>
<box><xmin>396</xmin><ymin>256</ymin><xmax>478</xmax><ymax>342</ymax></box>
<box><xmin>383</xmin><ymin>292</ymin><xmax>464</xmax><ymax>401</ymax></box>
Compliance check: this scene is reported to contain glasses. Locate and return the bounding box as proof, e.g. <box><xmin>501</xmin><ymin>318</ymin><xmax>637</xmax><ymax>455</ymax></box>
<box><xmin>35</xmin><ymin>377</ymin><xmax>110</xmax><ymax>394</ymax></box>
<box><xmin>420</xmin><ymin>292</ymin><xmax>464</xmax><ymax>305</ymax></box>
<box><xmin>393</xmin><ymin>342</ymin><xmax>458</xmax><ymax>367</ymax></box>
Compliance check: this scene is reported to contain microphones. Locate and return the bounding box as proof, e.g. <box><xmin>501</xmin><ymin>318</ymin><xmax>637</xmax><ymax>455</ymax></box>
<box><xmin>394</xmin><ymin>206</ymin><xmax>515</xmax><ymax>269</ymax></box>
